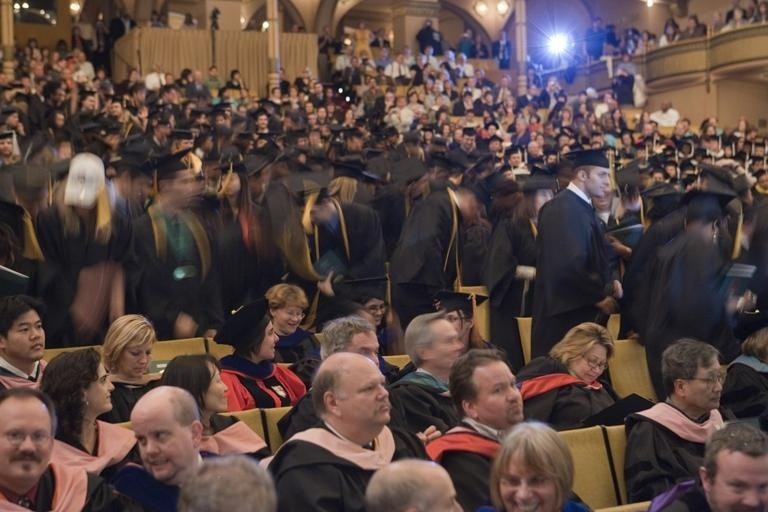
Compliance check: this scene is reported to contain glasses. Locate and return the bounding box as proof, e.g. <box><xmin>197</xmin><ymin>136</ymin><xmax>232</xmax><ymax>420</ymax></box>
<box><xmin>583</xmin><ymin>355</ymin><xmax>609</xmax><ymax>370</ymax></box>
<box><xmin>681</xmin><ymin>374</ymin><xmax>725</xmax><ymax>385</ymax></box>
<box><xmin>3</xmin><ymin>429</ymin><xmax>54</xmax><ymax>446</ymax></box>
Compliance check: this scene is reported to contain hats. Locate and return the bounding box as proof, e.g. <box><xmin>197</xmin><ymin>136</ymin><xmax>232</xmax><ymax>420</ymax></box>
<box><xmin>429</xmin><ymin>290</ymin><xmax>489</xmax><ymax>314</ymax></box>
<box><xmin>0</xmin><ymin>81</ymin><xmax>768</xmax><ymax>227</ymax></box>
<box><xmin>212</xmin><ymin>296</ymin><xmax>272</xmax><ymax>350</ymax></box>
<box><xmin>333</xmin><ymin>275</ymin><xmax>388</xmax><ymax>305</ymax></box>
<box><xmin>0</xmin><ymin>264</ymin><xmax>29</xmax><ymax>293</ymax></box>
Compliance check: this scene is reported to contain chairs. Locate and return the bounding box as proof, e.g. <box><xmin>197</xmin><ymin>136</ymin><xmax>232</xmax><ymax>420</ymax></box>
<box><xmin>43</xmin><ymin>280</ymin><xmax>662</xmax><ymax>512</ymax></box>
